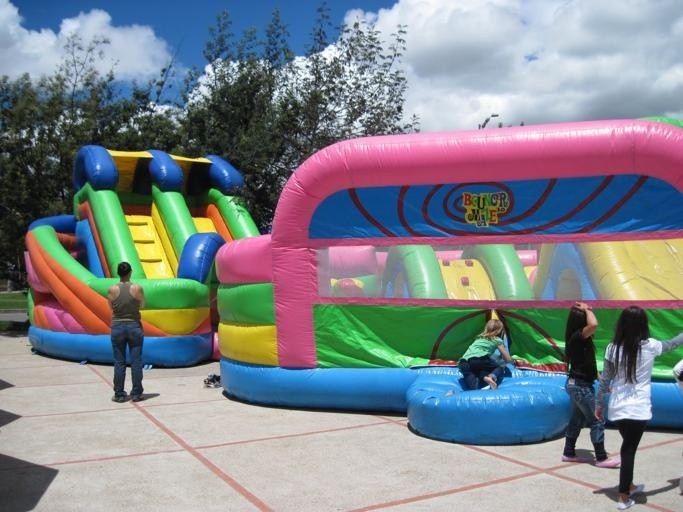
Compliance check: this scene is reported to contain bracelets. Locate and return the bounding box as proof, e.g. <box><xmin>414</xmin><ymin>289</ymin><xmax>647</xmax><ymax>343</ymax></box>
<box><xmin>585</xmin><ymin>307</ymin><xmax>593</xmax><ymax>311</ymax></box>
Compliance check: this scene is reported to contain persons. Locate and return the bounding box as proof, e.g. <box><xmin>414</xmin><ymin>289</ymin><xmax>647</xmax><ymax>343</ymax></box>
<box><xmin>458</xmin><ymin>319</ymin><xmax>512</xmax><ymax>390</ymax></box>
<box><xmin>594</xmin><ymin>305</ymin><xmax>683</xmax><ymax>510</ymax></box>
<box><xmin>561</xmin><ymin>301</ymin><xmax>621</xmax><ymax>468</ymax></box>
<box><xmin>107</xmin><ymin>262</ymin><xmax>146</xmax><ymax>403</ymax></box>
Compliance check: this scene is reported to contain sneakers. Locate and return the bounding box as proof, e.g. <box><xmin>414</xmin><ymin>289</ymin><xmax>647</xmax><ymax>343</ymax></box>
<box><xmin>617</xmin><ymin>483</ymin><xmax>644</xmax><ymax>510</ymax></box>
<box><xmin>594</xmin><ymin>458</ymin><xmax>620</xmax><ymax>469</ymax></box>
<box><xmin>112</xmin><ymin>394</ymin><xmax>125</xmax><ymax>402</ymax></box>
<box><xmin>203</xmin><ymin>373</ymin><xmax>221</xmax><ymax>388</ymax></box>
<box><xmin>562</xmin><ymin>455</ymin><xmax>588</xmax><ymax>463</ymax></box>
<box><xmin>131</xmin><ymin>393</ymin><xmax>145</xmax><ymax>402</ymax></box>
